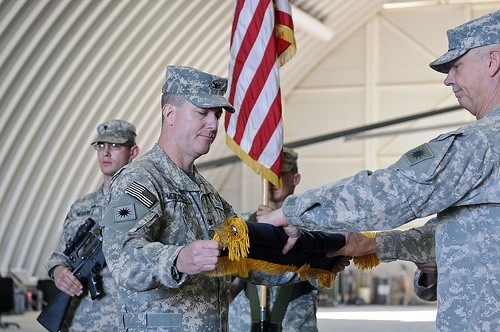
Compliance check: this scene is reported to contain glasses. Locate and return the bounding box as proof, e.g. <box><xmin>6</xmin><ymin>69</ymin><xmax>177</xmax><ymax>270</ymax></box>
<box><xmin>93</xmin><ymin>143</ymin><xmax>124</xmax><ymax>151</ymax></box>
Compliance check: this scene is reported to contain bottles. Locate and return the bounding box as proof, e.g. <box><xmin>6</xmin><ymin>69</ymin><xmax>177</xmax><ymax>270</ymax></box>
<box><xmin>338</xmin><ymin>259</ymin><xmax>375</xmax><ymax>304</ymax></box>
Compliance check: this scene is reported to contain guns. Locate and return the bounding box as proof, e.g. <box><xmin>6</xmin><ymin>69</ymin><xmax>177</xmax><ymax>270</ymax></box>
<box><xmin>37</xmin><ymin>218</ymin><xmax>112</xmax><ymax>332</ymax></box>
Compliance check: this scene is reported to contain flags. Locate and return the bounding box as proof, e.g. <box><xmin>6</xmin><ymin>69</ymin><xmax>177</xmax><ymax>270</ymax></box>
<box><xmin>224</xmin><ymin>0</ymin><xmax>296</xmax><ymax>189</ymax></box>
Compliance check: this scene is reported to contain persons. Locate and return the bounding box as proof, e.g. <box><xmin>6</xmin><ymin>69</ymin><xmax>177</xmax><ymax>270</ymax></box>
<box><xmin>103</xmin><ymin>65</ymin><xmax>242</xmax><ymax>332</ymax></box>
<box><xmin>238</xmin><ymin>147</ymin><xmax>318</xmax><ymax>332</ymax></box>
<box><xmin>41</xmin><ymin>120</ymin><xmax>139</xmax><ymax>332</ymax></box>
<box><xmin>256</xmin><ymin>10</ymin><xmax>500</xmax><ymax>332</ymax></box>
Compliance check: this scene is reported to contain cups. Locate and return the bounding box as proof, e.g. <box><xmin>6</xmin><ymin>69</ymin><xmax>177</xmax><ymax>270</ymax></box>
<box><xmin>375</xmin><ymin>277</ymin><xmax>391</xmax><ymax>304</ymax></box>
<box><xmin>12</xmin><ymin>288</ymin><xmax>42</xmax><ymax>315</ymax></box>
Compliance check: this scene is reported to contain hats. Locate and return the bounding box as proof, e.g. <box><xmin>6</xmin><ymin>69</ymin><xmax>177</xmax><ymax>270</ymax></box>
<box><xmin>161</xmin><ymin>64</ymin><xmax>237</xmax><ymax>114</ymax></box>
<box><xmin>428</xmin><ymin>8</ymin><xmax>500</xmax><ymax>75</ymax></box>
<box><xmin>278</xmin><ymin>146</ymin><xmax>300</xmax><ymax>174</ymax></box>
<box><xmin>89</xmin><ymin>119</ymin><xmax>138</xmax><ymax>146</ymax></box>
